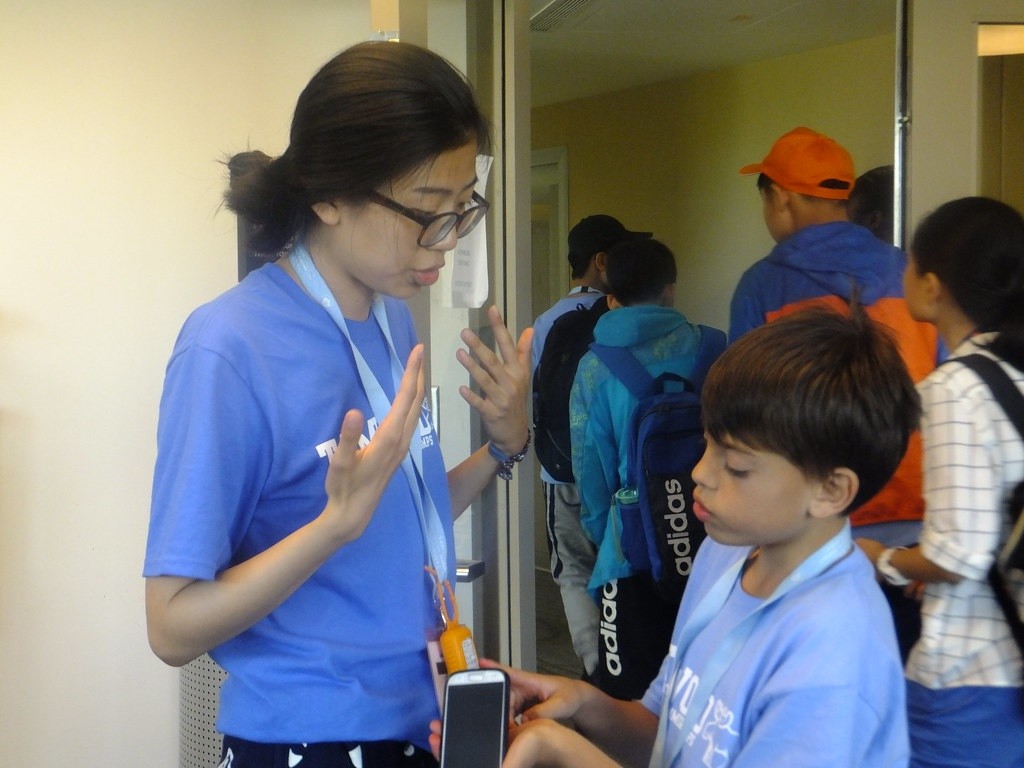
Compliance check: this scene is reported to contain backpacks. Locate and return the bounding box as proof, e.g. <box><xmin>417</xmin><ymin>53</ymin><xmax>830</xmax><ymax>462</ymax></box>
<box><xmin>533</xmin><ymin>298</ymin><xmax>610</xmax><ymax>484</ymax></box>
<box><xmin>588</xmin><ymin>324</ymin><xmax>727</xmax><ymax>615</ymax></box>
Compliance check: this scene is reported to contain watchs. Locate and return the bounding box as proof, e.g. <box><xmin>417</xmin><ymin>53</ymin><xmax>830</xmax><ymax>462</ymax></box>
<box><xmin>876</xmin><ymin>546</ymin><xmax>914</xmax><ymax>586</ymax></box>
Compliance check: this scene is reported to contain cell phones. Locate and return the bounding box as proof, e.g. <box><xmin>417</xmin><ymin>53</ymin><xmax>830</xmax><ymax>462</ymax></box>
<box><xmin>441</xmin><ymin>667</ymin><xmax>510</xmax><ymax>768</ymax></box>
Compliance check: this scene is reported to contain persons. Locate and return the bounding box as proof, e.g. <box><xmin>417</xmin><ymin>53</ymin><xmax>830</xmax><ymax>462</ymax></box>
<box><xmin>850</xmin><ymin>197</ymin><xmax>1024</xmax><ymax>768</ymax></box>
<box><xmin>141</xmin><ymin>42</ymin><xmax>535</xmax><ymax>768</ymax></box>
<box><xmin>527</xmin><ymin>125</ymin><xmax>950</xmax><ymax>702</ymax></box>
<box><xmin>428</xmin><ymin>303</ymin><xmax>920</xmax><ymax>768</ymax></box>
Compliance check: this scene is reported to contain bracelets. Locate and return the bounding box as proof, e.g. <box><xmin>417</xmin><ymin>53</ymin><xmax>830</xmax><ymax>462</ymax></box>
<box><xmin>487</xmin><ymin>427</ymin><xmax>533</xmax><ymax>482</ymax></box>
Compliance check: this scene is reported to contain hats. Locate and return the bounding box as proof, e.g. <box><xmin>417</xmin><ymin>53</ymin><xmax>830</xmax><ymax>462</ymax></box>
<box><xmin>568</xmin><ymin>215</ymin><xmax>653</xmax><ymax>265</ymax></box>
<box><xmin>740</xmin><ymin>127</ymin><xmax>855</xmax><ymax>200</ymax></box>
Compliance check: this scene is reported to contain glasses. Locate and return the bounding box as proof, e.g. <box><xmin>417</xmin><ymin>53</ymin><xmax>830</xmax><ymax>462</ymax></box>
<box><xmin>366</xmin><ymin>190</ymin><xmax>490</xmax><ymax>247</ymax></box>
